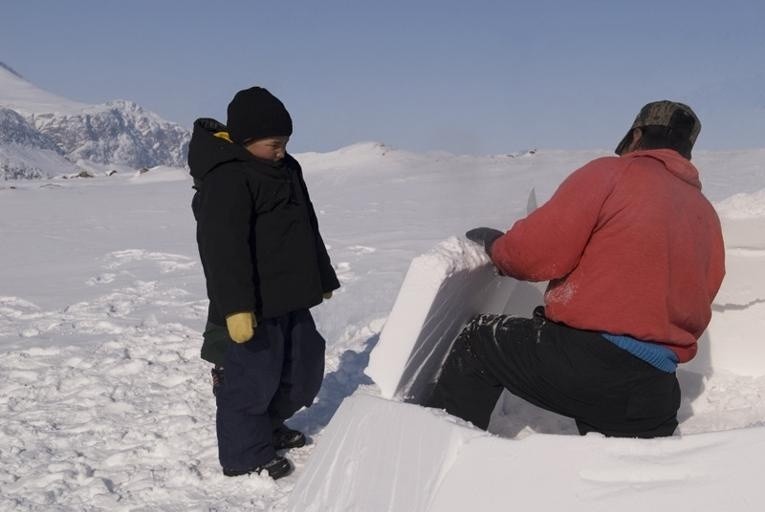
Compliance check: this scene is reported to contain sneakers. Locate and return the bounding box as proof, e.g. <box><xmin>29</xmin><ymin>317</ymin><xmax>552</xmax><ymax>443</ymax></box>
<box><xmin>222</xmin><ymin>454</ymin><xmax>291</xmax><ymax>480</ymax></box>
<box><xmin>272</xmin><ymin>423</ymin><xmax>307</xmax><ymax>450</ymax></box>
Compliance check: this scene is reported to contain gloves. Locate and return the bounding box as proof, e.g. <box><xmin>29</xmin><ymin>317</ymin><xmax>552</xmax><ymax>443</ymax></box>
<box><xmin>466</xmin><ymin>225</ymin><xmax>508</xmax><ymax>278</ymax></box>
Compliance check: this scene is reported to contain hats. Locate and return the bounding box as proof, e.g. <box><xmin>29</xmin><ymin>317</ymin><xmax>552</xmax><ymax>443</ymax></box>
<box><xmin>613</xmin><ymin>100</ymin><xmax>702</xmax><ymax>157</ymax></box>
<box><xmin>224</xmin><ymin>86</ymin><xmax>293</xmax><ymax>146</ymax></box>
<box><xmin>224</xmin><ymin>311</ymin><xmax>258</xmax><ymax>344</ymax></box>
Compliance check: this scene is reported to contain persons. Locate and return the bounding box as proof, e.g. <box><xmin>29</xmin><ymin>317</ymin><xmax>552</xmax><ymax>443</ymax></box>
<box><xmin>185</xmin><ymin>85</ymin><xmax>340</xmax><ymax>480</ymax></box>
<box><xmin>430</xmin><ymin>101</ymin><xmax>726</xmax><ymax>438</ymax></box>
